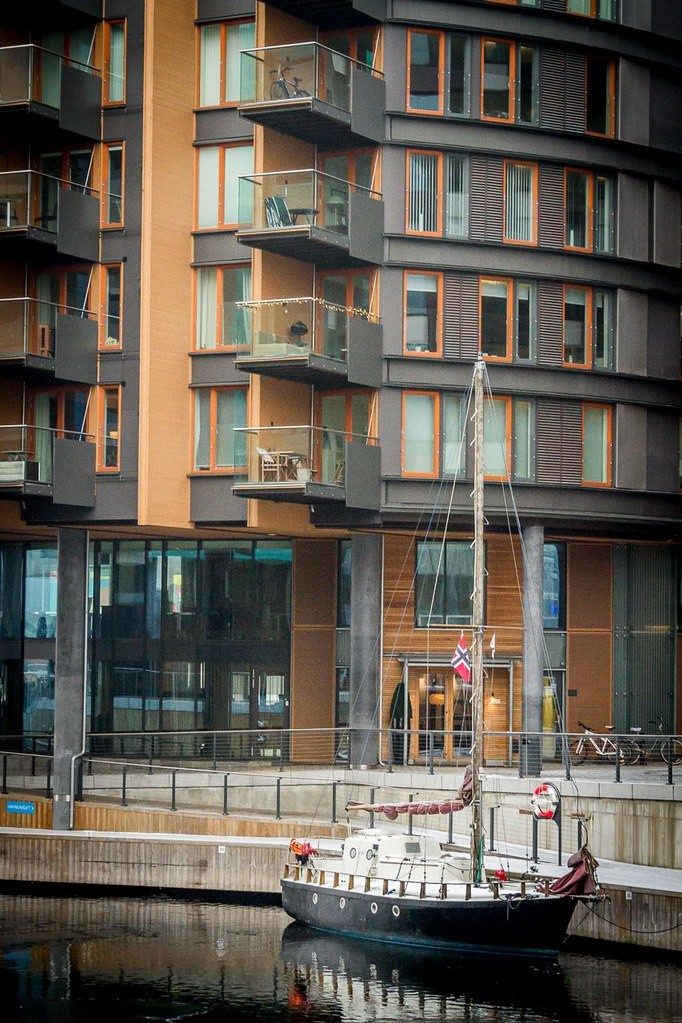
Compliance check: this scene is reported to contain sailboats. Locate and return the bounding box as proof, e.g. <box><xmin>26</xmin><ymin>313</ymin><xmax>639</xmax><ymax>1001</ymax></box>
<box><xmin>278</xmin><ymin>350</ymin><xmax>611</xmax><ymax>958</ymax></box>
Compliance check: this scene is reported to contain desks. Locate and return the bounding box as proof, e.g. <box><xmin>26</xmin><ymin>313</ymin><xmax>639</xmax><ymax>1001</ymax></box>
<box><xmin>260</xmin><ymin>450</ymin><xmax>296</xmax><ymax>482</ymax></box>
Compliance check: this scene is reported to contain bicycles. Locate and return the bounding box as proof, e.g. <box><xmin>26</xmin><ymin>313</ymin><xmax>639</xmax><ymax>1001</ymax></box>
<box><xmin>617</xmin><ymin>713</ymin><xmax>682</xmax><ymax>766</ymax></box>
<box><xmin>568</xmin><ymin>720</ymin><xmax>633</xmax><ymax>766</ymax></box>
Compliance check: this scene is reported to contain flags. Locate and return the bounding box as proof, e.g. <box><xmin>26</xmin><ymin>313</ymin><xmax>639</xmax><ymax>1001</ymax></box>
<box><xmin>451</xmin><ymin>634</ymin><xmax>471</xmax><ymax>683</ymax></box>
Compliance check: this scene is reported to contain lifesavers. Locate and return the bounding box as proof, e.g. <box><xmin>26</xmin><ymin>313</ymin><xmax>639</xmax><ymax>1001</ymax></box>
<box><xmin>531</xmin><ymin>784</ymin><xmax>559</xmax><ymax>820</ymax></box>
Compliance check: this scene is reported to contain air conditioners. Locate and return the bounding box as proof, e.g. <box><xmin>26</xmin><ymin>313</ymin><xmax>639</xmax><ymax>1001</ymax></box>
<box><xmin>38</xmin><ymin>323</ymin><xmax>55</xmax><ymax>354</ymax></box>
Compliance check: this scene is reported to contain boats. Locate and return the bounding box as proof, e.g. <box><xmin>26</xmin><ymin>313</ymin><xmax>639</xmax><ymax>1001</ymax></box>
<box><xmin>278</xmin><ymin>922</ymin><xmax>595</xmax><ymax>1023</ymax></box>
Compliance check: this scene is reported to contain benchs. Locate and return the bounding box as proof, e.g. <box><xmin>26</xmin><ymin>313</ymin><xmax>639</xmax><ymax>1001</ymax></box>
<box><xmin>289</xmin><ymin>208</ymin><xmax>319</xmax><ymax>225</ymax></box>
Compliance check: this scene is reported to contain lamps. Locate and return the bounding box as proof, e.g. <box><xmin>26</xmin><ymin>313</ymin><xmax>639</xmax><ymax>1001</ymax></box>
<box><xmin>326</xmin><ymin>195</ymin><xmax>346</xmax><ymax>226</ymax></box>
<box><xmin>429</xmin><ymin>673</ymin><xmax>445</xmax><ymax>706</ymax></box>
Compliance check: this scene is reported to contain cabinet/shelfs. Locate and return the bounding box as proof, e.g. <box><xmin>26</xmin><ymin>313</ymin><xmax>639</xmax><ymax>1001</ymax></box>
<box><xmin>0</xmin><ymin>461</ymin><xmax>39</xmax><ymax>483</ymax></box>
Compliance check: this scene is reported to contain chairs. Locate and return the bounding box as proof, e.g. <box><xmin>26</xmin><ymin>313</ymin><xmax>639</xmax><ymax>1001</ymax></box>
<box><xmin>0</xmin><ymin>202</ymin><xmax>20</xmax><ymax>227</ymax></box>
<box><xmin>34</xmin><ymin>201</ymin><xmax>57</xmax><ymax>230</ymax></box>
<box><xmin>262</xmin><ymin>452</ymin><xmax>311</xmax><ymax>483</ymax></box>
